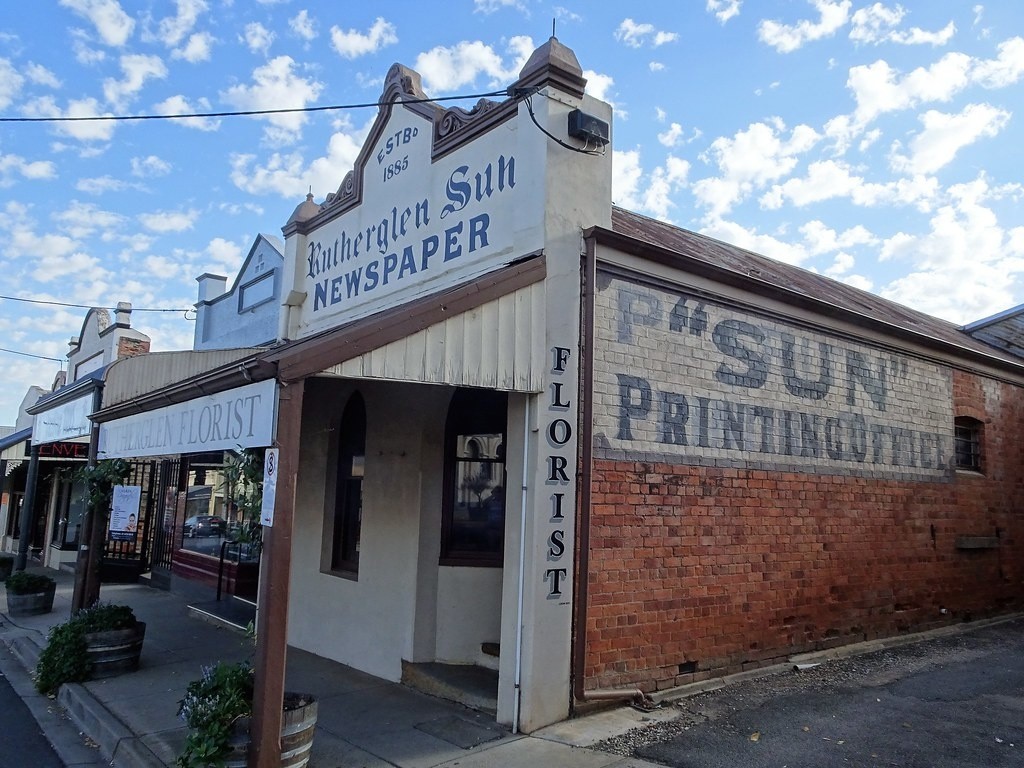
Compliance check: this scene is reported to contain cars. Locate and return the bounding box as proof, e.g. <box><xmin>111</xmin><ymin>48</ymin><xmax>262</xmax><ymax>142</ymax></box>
<box><xmin>184</xmin><ymin>515</ymin><xmax>227</xmax><ymax>538</ymax></box>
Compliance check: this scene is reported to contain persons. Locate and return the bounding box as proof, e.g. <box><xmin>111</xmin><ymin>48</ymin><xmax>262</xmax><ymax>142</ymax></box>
<box><xmin>124</xmin><ymin>514</ymin><xmax>137</xmax><ymax>531</ymax></box>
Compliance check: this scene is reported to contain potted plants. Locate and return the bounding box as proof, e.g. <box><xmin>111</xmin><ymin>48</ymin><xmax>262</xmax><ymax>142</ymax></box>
<box><xmin>4</xmin><ymin>571</ymin><xmax>57</xmax><ymax>617</ymax></box>
<box><xmin>461</xmin><ymin>469</ymin><xmax>494</xmax><ymax>521</ymax></box>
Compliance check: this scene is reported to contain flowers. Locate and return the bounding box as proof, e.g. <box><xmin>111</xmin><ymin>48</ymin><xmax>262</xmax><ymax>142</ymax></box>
<box><xmin>33</xmin><ymin>598</ymin><xmax>136</xmax><ymax>697</ymax></box>
<box><xmin>175</xmin><ymin>619</ymin><xmax>256</xmax><ymax>768</ymax></box>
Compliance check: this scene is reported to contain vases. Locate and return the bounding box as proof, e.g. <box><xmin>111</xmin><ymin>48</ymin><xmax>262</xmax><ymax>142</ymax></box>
<box><xmin>209</xmin><ymin>692</ymin><xmax>319</xmax><ymax>768</ymax></box>
<box><xmin>79</xmin><ymin>621</ymin><xmax>146</xmax><ymax>682</ymax></box>
